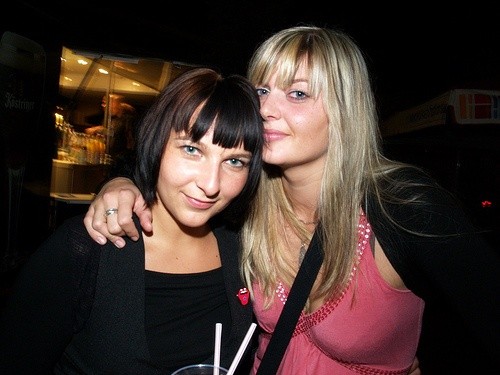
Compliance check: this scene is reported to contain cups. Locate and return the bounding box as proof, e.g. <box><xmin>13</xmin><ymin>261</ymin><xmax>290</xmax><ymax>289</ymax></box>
<box><xmin>171</xmin><ymin>364</ymin><xmax>233</xmax><ymax>375</ymax></box>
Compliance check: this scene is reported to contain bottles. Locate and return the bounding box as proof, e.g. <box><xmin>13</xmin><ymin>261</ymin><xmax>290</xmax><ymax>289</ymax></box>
<box><xmin>67</xmin><ymin>132</ymin><xmax>107</xmax><ymax>165</ymax></box>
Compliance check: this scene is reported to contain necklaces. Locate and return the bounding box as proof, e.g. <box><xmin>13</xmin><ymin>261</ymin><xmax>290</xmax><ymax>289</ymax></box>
<box><xmin>275</xmin><ymin>188</ymin><xmax>339</xmax><ymax>279</ymax></box>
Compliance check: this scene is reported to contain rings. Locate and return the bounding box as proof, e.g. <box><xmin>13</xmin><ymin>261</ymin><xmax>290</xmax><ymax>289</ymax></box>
<box><xmin>105</xmin><ymin>206</ymin><xmax>118</xmax><ymax>216</ymax></box>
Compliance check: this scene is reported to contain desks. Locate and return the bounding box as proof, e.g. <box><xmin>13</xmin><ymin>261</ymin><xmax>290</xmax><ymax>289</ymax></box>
<box><xmin>51</xmin><ymin>192</ymin><xmax>95</xmax><ymax>225</ymax></box>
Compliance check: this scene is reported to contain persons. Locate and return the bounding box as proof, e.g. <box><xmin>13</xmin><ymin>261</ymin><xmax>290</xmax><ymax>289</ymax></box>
<box><xmin>83</xmin><ymin>92</ymin><xmax>139</xmax><ymax>179</ymax></box>
<box><xmin>84</xmin><ymin>26</ymin><xmax>500</xmax><ymax>375</ymax></box>
<box><xmin>0</xmin><ymin>68</ymin><xmax>261</xmax><ymax>375</ymax></box>
